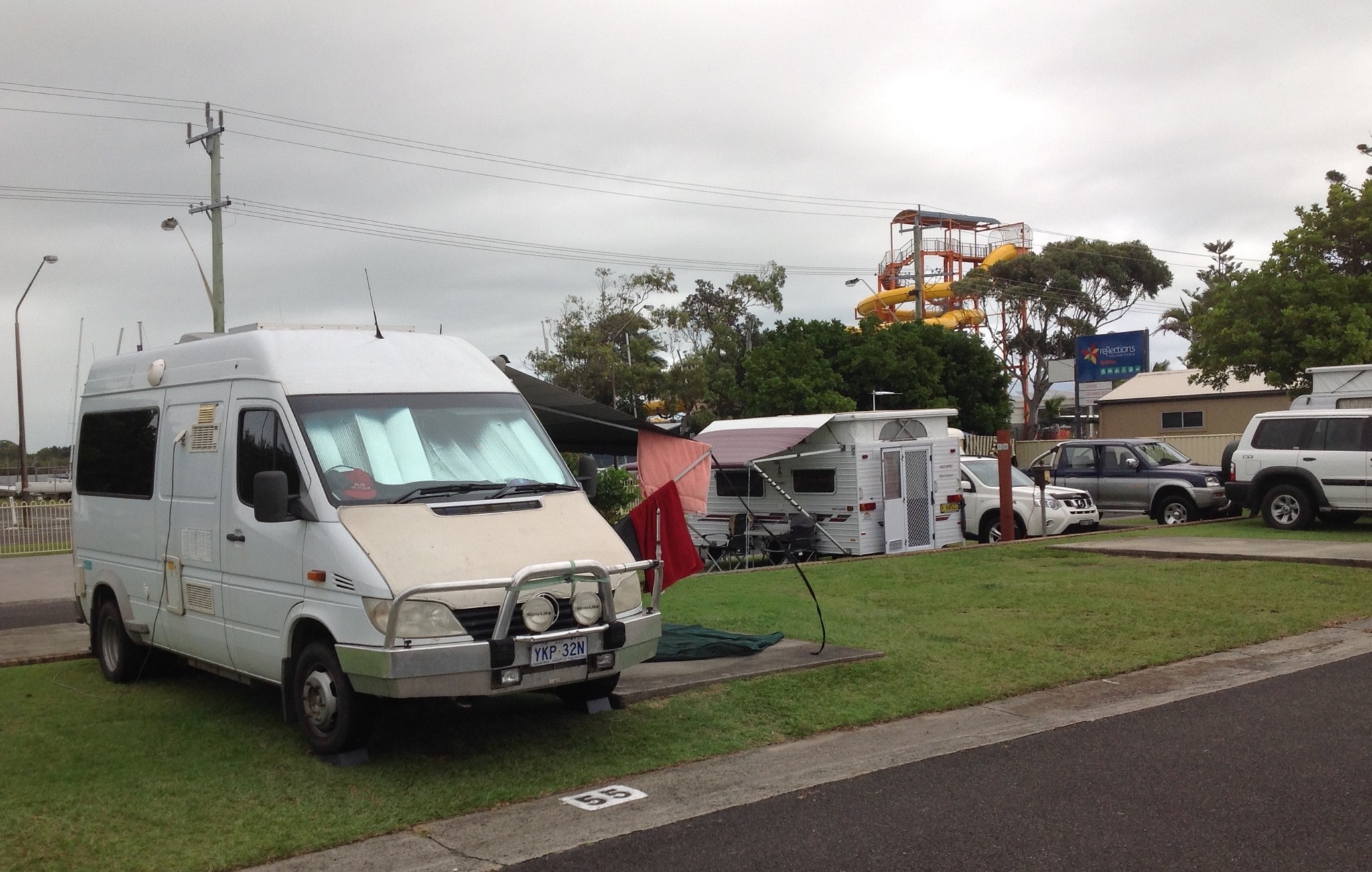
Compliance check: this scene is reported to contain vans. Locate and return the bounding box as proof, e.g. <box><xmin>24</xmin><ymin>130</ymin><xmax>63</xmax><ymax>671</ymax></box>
<box><xmin>68</xmin><ymin>324</ymin><xmax>667</xmax><ymax>758</ymax></box>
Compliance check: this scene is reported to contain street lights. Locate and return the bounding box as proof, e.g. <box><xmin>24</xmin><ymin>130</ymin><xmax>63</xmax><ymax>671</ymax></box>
<box><xmin>14</xmin><ymin>256</ymin><xmax>58</xmax><ymax>527</ymax></box>
<box><xmin>844</xmin><ymin>277</ymin><xmax>900</xmax><ymax>324</ymax></box>
<box><xmin>161</xmin><ymin>218</ymin><xmax>212</xmax><ymax>313</ymax></box>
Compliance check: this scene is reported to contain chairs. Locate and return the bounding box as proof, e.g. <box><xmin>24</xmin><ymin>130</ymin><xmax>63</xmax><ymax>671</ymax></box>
<box><xmin>1073</xmin><ymin>446</ymin><xmax>1135</xmax><ymax>471</ymax></box>
<box><xmin>761</xmin><ymin>512</ymin><xmax>818</xmax><ymax>566</ymax></box>
<box><xmin>698</xmin><ymin>513</ymin><xmax>755</xmax><ymax>574</ymax></box>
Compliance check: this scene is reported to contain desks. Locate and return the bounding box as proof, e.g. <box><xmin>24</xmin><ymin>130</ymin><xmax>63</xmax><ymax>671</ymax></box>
<box><xmin>742</xmin><ymin>528</ymin><xmax>792</xmax><ymax>570</ymax></box>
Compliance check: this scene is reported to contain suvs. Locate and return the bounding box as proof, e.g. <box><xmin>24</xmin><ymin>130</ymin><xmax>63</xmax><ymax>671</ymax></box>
<box><xmin>1221</xmin><ymin>409</ymin><xmax>1372</xmax><ymax>526</ymax></box>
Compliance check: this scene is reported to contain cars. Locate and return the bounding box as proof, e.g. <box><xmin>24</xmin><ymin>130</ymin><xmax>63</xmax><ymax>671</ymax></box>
<box><xmin>959</xmin><ymin>456</ymin><xmax>1100</xmax><ymax>546</ymax></box>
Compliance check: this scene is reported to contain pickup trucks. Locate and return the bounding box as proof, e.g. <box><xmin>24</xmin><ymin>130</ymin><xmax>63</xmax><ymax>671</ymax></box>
<box><xmin>1017</xmin><ymin>437</ymin><xmax>1231</xmax><ymax>526</ymax></box>
